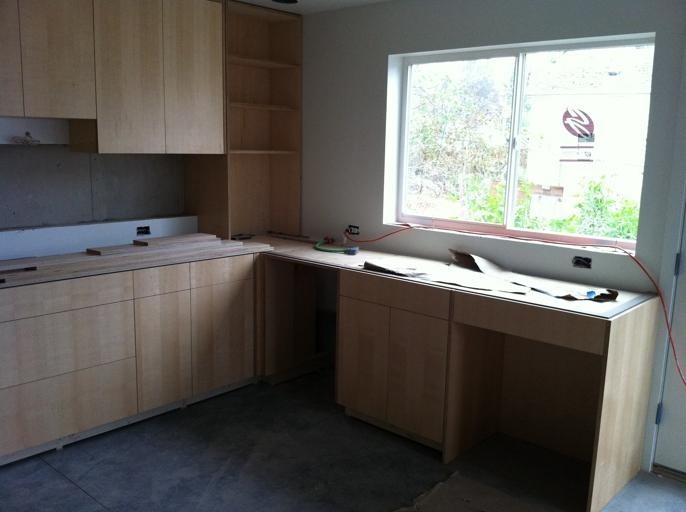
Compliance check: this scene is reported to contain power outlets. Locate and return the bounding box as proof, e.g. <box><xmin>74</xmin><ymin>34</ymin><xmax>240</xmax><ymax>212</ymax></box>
<box><xmin>348</xmin><ymin>225</ymin><xmax>360</xmax><ymax>235</ymax></box>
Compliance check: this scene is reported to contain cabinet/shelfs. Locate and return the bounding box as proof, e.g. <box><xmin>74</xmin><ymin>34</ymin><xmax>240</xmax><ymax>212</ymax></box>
<box><xmin>131</xmin><ymin>254</ymin><xmax>256</xmax><ymax>415</ymax></box>
<box><xmin>443</xmin><ymin>290</ymin><xmax>661</xmax><ymax>512</ymax></box>
<box><xmin>2</xmin><ymin>270</ymin><xmax>138</xmax><ymax>468</ymax></box>
<box><xmin>0</xmin><ymin>0</ymin><xmax>97</xmax><ymax>121</ymax></box>
<box><xmin>183</xmin><ymin>0</ymin><xmax>302</xmax><ymax>241</ymax></box>
<box><xmin>335</xmin><ymin>269</ymin><xmax>450</xmax><ymax>451</ymax></box>
<box><xmin>69</xmin><ymin>0</ymin><xmax>228</xmax><ymax>159</ymax></box>
